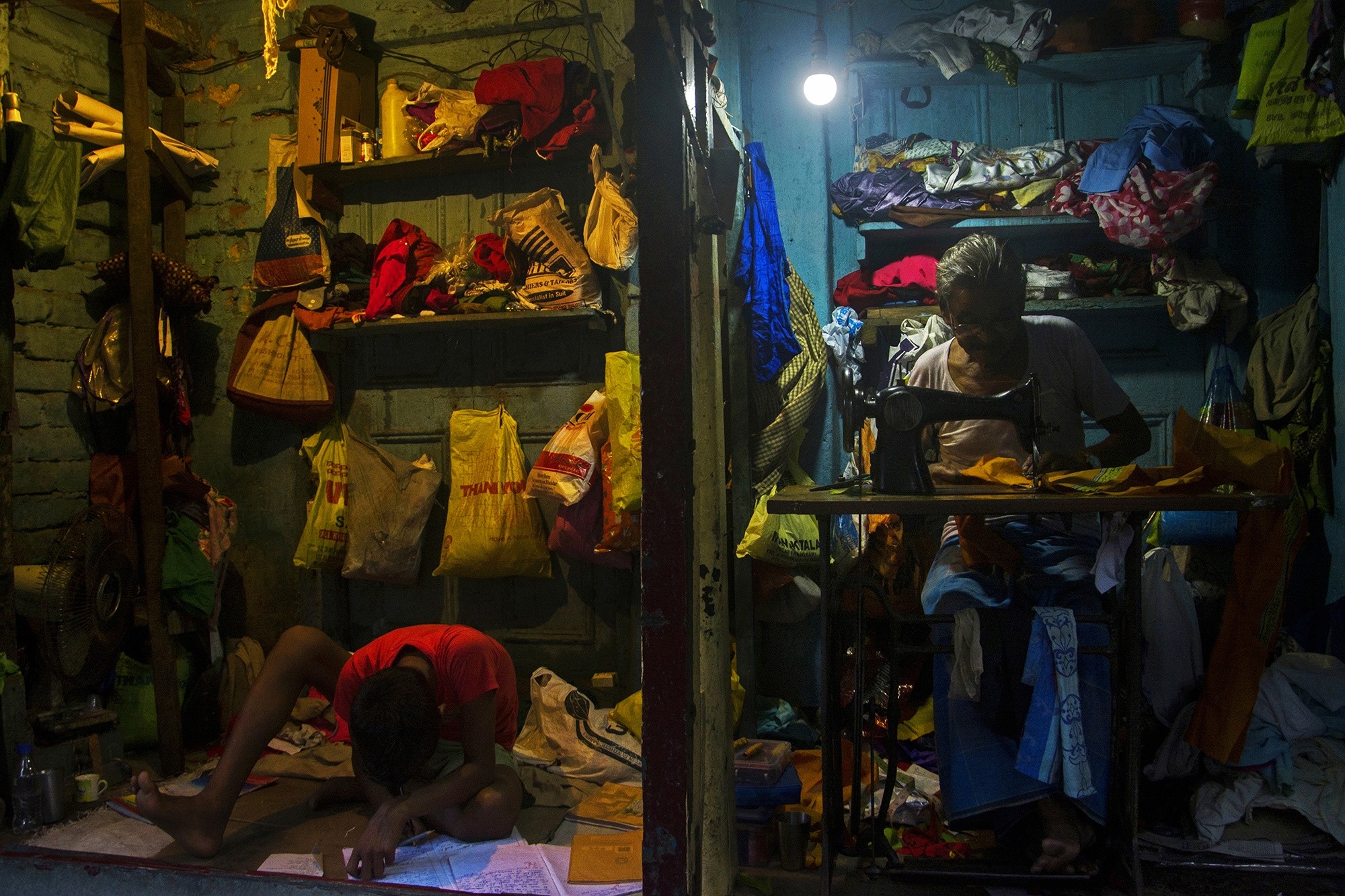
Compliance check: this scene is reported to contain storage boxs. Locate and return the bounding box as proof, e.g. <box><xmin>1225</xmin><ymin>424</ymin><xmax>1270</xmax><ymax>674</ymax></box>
<box><xmin>732</xmin><ymin>738</ymin><xmax>802</xmax><ymax>865</ymax></box>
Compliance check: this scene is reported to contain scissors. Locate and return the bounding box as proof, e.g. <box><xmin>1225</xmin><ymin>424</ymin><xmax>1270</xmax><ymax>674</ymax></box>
<box><xmin>809</xmin><ymin>473</ymin><xmax>871</xmax><ymax>492</ymax></box>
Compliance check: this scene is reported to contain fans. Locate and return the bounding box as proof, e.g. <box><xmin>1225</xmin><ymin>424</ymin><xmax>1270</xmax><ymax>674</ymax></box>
<box><xmin>11</xmin><ymin>504</ymin><xmax>141</xmax><ymax>735</ymax></box>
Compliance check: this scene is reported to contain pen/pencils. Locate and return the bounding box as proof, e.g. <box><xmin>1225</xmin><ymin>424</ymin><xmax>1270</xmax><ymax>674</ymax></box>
<box><xmin>396</xmin><ymin>829</ymin><xmax>435</xmax><ymax>846</ymax></box>
<box><xmin>399</xmin><ymin>788</ymin><xmax>416</xmax><ymax>847</ymax></box>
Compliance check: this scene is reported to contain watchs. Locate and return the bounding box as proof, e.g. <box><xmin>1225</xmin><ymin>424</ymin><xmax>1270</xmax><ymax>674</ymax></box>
<box><xmin>1078</xmin><ymin>450</ymin><xmax>1102</xmax><ymax>469</ymax></box>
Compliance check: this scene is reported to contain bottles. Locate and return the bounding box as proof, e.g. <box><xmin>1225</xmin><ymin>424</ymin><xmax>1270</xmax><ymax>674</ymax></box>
<box><xmin>11</xmin><ymin>742</ymin><xmax>43</xmax><ymax>835</ymax></box>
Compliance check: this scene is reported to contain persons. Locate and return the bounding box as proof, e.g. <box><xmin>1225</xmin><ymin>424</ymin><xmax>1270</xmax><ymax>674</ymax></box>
<box><xmin>902</xmin><ymin>231</ymin><xmax>1153</xmax><ymax>873</ymax></box>
<box><xmin>131</xmin><ymin>622</ymin><xmax>522</xmax><ymax>880</ymax></box>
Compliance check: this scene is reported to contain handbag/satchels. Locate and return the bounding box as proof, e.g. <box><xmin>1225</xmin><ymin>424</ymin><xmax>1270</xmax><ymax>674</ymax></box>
<box><xmin>222</xmin><ymin>292</ymin><xmax>341</xmax><ymax>415</ymax></box>
<box><xmin>363</xmin><ymin>218</ymin><xmax>458</xmax><ymax>321</ymax></box>
<box><xmin>491</xmin><ymin>186</ymin><xmax>604</xmax><ymax>309</ymax></box>
<box><xmin>406</xmin><ymin>84</ymin><xmax>491</xmax><ymax>151</ymax></box>
<box><xmin>616</xmin><ymin>654</ymin><xmax>744</xmax><ymax>756</ymax></box>
<box><xmin>254</xmin><ymin>126</ymin><xmax>331</xmax><ymax>285</ymax></box>
<box><xmin>583</xmin><ymin>142</ymin><xmax>639</xmax><ymax>271</ymax></box>
<box><xmin>430</xmin><ymin>404</ymin><xmax>552</xmax><ymax>581</ymax></box>
<box><xmin>339</xmin><ymin>420</ymin><xmax>442</xmax><ymax>580</ymax></box>
<box><xmin>294</xmin><ymin>419</ymin><xmax>358</xmax><ymax>572</ymax></box>
<box><xmin>829</xmin><ymin>451</ymin><xmax>870</xmax><ymax>576</ymax></box>
<box><xmin>512</xmin><ymin>667</ymin><xmax>645</xmax><ymax>785</ymax></box>
<box><xmin>521</xmin><ymin>349</ymin><xmax>642</xmax><ymax>567</ymax></box>
<box><xmin>420</xmin><ymin>232</ymin><xmax>492</xmax><ymax>287</ymax></box>
<box><xmin>739</xmin><ymin>455</ymin><xmax>837</xmax><ymax>571</ymax></box>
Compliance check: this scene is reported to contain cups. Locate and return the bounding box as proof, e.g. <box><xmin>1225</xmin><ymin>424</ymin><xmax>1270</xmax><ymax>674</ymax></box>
<box><xmin>777</xmin><ymin>811</ymin><xmax>812</xmax><ymax>871</ymax></box>
<box><xmin>82</xmin><ymin>693</ymin><xmax>103</xmax><ymax>723</ymax></box>
<box><xmin>75</xmin><ymin>773</ymin><xmax>108</xmax><ymax>803</ymax></box>
<box><xmin>40</xmin><ymin>765</ymin><xmax>65</xmax><ymax>825</ymax></box>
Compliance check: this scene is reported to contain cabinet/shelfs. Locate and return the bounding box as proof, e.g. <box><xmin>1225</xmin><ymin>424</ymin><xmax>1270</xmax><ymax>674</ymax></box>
<box><xmin>305</xmin><ymin>146</ymin><xmax>606</xmax><ymax>351</ymax></box>
<box><xmin>857</xmin><ymin>41</ymin><xmax>1217</xmax><ymax>349</ymax></box>
